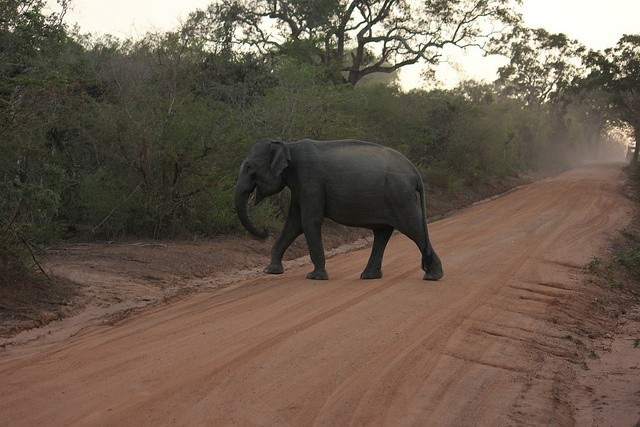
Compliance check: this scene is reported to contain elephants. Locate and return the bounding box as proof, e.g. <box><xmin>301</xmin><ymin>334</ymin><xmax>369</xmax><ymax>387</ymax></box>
<box><xmin>234</xmin><ymin>137</ymin><xmax>444</xmax><ymax>280</ymax></box>
